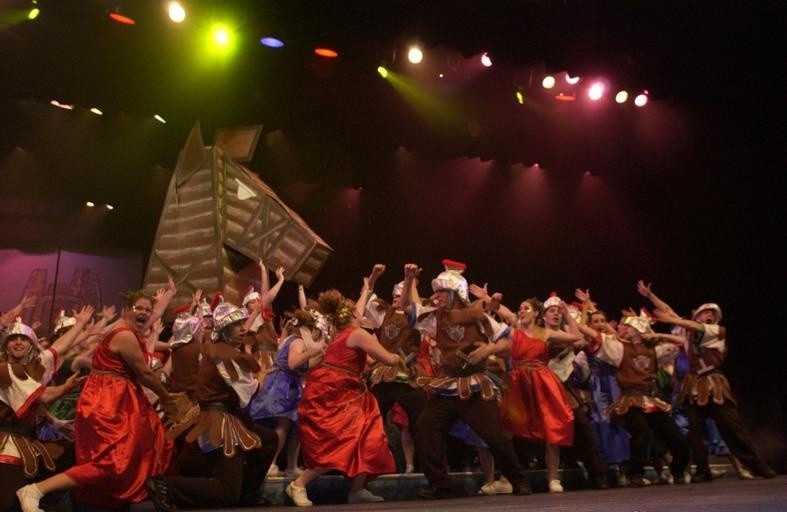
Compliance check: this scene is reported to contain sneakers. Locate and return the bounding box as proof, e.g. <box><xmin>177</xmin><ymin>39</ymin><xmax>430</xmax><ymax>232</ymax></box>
<box><xmin>598</xmin><ymin>464</ymin><xmax>775</xmax><ymax>489</ymax></box>
<box><xmin>265</xmin><ymin>464</ymin><xmax>313</xmax><ymax>507</ymax></box>
<box><xmin>15</xmin><ymin>485</ymin><xmax>45</xmax><ymax>512</ymax></box>
<box><xmin>146</xmin><ymin>479</ymin><xmax>179</xmax><ymax>512</ymax></box>
<box><xmin>347</xmin><ymin>488</ymin><xmax>384</xmax><ymax>504</ymax></box>
<box><xmin>417</xmin><ymin>463</ymin><xmax>532</xmax><ymax>499</ymax></box>
<box><xmin>549</xmin><ymin>479</ymin><xmax>564</xmax><ymax>493</ymax></box>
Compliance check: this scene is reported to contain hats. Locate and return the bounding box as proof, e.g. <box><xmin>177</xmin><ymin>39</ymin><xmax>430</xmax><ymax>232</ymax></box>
<box><xmin>691</xmin><ymin>303</ymin><xmax>723</xmax><ymax>324</ymax></box>
<box><xmin>170</xmin><ymin>285</ymin><xmax>262</xmax><ymax>350</ymax></box>
<box><xmin>542</xmin><ymin>291</ymin><xmax>588</xmax><ymax>332</ymax></box>
<box><xmin>624</xmin><ymin>306</ymin><xmax>658</xmax><ymax>344</ymax></box>
<box><xmin>392</xmin><ymin>259</ymin><xmax>471</xmax><ymax>307</ymax></box>
<box><xmin>0</xmin><ymin>309</ymin><xmax>76</xmax><ymax>350</ymax></box>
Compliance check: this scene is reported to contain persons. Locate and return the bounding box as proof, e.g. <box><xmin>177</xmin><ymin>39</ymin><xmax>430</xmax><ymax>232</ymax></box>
<box><xmin>0</xmin><ymin>260</ymin><xmax>780</xmax><ymax>511</ymax></box>
